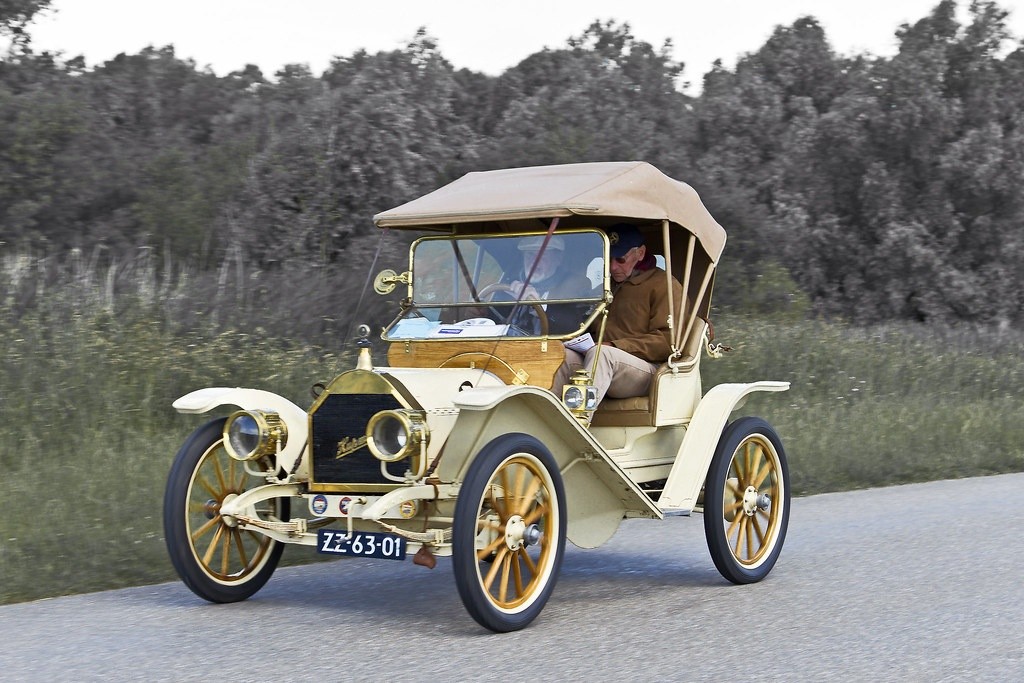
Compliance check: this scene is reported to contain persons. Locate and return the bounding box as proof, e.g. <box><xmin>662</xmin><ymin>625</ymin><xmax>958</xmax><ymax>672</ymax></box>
<box><xmin>466</xmin><ymin>234</ymin><xmax>587</xmax><ymax>337</ymax></box>
<box><xmin>551</xmin><ymin>223</ymin><xmax>691</xmax><ymax>426</ymax></box>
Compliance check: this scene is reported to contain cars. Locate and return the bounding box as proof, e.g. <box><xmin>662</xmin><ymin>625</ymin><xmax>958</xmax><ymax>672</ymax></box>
<box><xmin>163</xmin><ymin>161</ymin><xmax>793</xmax><ymax>634</ymax></box>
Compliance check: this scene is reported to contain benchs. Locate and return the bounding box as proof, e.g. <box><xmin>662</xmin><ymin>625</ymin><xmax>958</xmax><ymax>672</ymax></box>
<box><xmin>588</xmin><ymin>314</ymin><xmax>709</xmax><ymax>428</ymax></box>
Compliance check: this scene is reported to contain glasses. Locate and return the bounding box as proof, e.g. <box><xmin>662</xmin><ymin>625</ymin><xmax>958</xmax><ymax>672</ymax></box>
<box><xmin>610</xmin><ymin>250</ymin><xmax>635</xmax><ymax>263</ymax></box>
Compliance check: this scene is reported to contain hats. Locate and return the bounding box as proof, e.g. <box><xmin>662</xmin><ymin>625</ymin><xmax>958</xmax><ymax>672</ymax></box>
<box><xmin>604</xmin><ymin>223</ymin><xmax>644</xmax><ymax>257</ymax></box>
<box><xmin>518</xmin><ymin>234</ymin><xmax>565</xmax><ymax>252</ymax></box>
